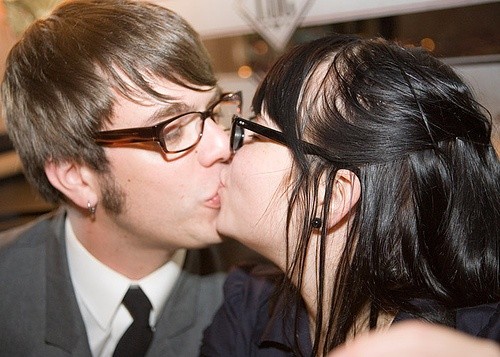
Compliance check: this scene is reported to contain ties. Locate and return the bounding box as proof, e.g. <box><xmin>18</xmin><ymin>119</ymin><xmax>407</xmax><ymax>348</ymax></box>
<box><xmin>112</xmin><ymin>284</ymin><xmax>157</xmax><ymax>357</ymax></box>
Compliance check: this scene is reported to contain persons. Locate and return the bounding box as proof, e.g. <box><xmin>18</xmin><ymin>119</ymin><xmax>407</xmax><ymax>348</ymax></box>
<box><xmin>216</xmin><ymin>33</ymin><xmax>500</xmax><ymax>357</ymax></box>
<box><xmin>0</xmin><ymin>0</ymin><xmax>315</xmax><ymax>357</ymax></box>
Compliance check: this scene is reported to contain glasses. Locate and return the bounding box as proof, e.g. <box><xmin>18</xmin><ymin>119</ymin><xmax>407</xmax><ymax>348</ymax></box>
<box><xmin>229</xmin><ymin>115</ymin><xmax>361</xmax><ymax>176</ymax></box>
<box><xmin>90</xmin><ymin>90</ymin><xmax>243</xmax><ymax>154</ymax></box>
